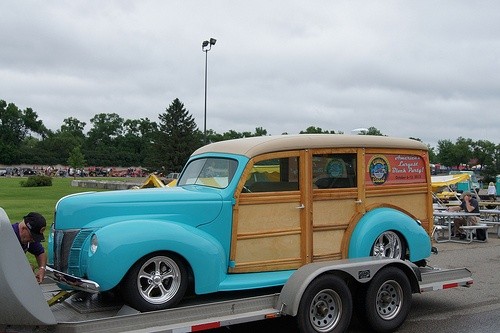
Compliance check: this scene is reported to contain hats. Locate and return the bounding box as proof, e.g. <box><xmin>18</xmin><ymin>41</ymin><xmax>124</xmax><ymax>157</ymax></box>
<box><xmin>460</xmin><ymin>191</ymin><xmax>470</xmax><ymax>199</ymax></box>
<box><xmin>23</xmin><ymin>212</ymin><xmax>46</xmax><ymax>240</ymax></box>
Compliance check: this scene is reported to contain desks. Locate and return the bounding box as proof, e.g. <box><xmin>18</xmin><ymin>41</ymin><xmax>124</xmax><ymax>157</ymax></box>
<box><xmin>479</xmin><ymin>210</ymin><xmax>500</xmax><ymax>238</ymax></box>
<box><xmin>433</xmin><ymin>212</ymin><xmax>480</xmax><ymax>243</ymax></box>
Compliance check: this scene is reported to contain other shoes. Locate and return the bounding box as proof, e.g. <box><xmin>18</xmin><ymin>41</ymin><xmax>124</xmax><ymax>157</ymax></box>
<box><xmin>468</xmin><ymin>232</ymin><xmax>476</xmax><ymax>239</ymax></box>
<box><xmin>459</xmin><ymin>233</ymin><xmax>466</xmax><ymax>240</ymax></box>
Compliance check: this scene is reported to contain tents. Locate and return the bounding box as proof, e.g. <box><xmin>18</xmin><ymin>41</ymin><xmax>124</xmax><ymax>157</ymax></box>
<box><xmin>430</xmin><ymin>175</ymin><xmax>481</xmax><ymax>210</ymax></box>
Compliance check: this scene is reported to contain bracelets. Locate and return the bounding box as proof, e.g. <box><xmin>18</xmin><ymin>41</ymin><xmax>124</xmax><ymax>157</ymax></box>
<box><xmin>40</xmin><ymin>266</ymin><xmax>46</xmax><ymax>271</ymax></box>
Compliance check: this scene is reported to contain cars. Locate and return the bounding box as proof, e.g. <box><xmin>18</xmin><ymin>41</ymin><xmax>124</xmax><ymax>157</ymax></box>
<box><xmin>40</xmin><ymin>133</ymin><xmax>438</xmax><ymax>315</ymax></box>
<box><xmin>159</xmin><ymin>173</ymin><xmax>181</xmax><ymax>185</ymax></box>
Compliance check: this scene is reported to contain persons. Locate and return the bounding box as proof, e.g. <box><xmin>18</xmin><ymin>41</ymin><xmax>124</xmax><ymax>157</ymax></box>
<box><xmin>487</xmin><ymin>182</ymin><xmax>496</xmax><ymax>196</ymax></box>
<box><xmin>45</xmin><ymin>166</ymin><xmax>137</xmax><ymax>177</ymax></box>
<box><xmin>447</xmin><ymin>191</ymin><xmax>480</xmax><ymax>239</ymax></box>
<box><xmin>11</xmin><ymin>212</ymin><xmax>47</xmax><ymax>284</ymax></box>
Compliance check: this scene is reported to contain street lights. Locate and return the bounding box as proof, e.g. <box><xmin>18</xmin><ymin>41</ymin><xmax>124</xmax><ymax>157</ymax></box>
<box><xmin>201</xmin><ymin>37</ymin><xmax>217</xmax><ymax>132</ymax></box>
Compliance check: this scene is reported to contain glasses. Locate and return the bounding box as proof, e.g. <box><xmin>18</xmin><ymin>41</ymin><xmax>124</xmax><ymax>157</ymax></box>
<box><xmin>26</xmin><ymin>230</ymin><xmax>45</xmax><ymax>242</ymax></box>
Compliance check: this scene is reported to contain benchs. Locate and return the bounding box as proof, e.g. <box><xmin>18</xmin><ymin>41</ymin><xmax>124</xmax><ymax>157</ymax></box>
<box><xmin>72</xmin><ymin>179</ymin><xmax>142</xmax><ymax>190</ymax></box>
<box><xmin>432</xmin><ymin>219</ymin><xmax>500</xmax><ymax>243</ymax></box>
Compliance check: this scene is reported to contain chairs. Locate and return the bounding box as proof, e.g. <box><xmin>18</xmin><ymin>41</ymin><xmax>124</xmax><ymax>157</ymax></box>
<box><xmin>250</xmin><ymin>182</ymin><xmax>299</xmax><ymax>192</ymax></box>
<box><xmin>315</xmin><ymin>178</ymin><xmax>357</xmax><ymax>190</ymax></box>
